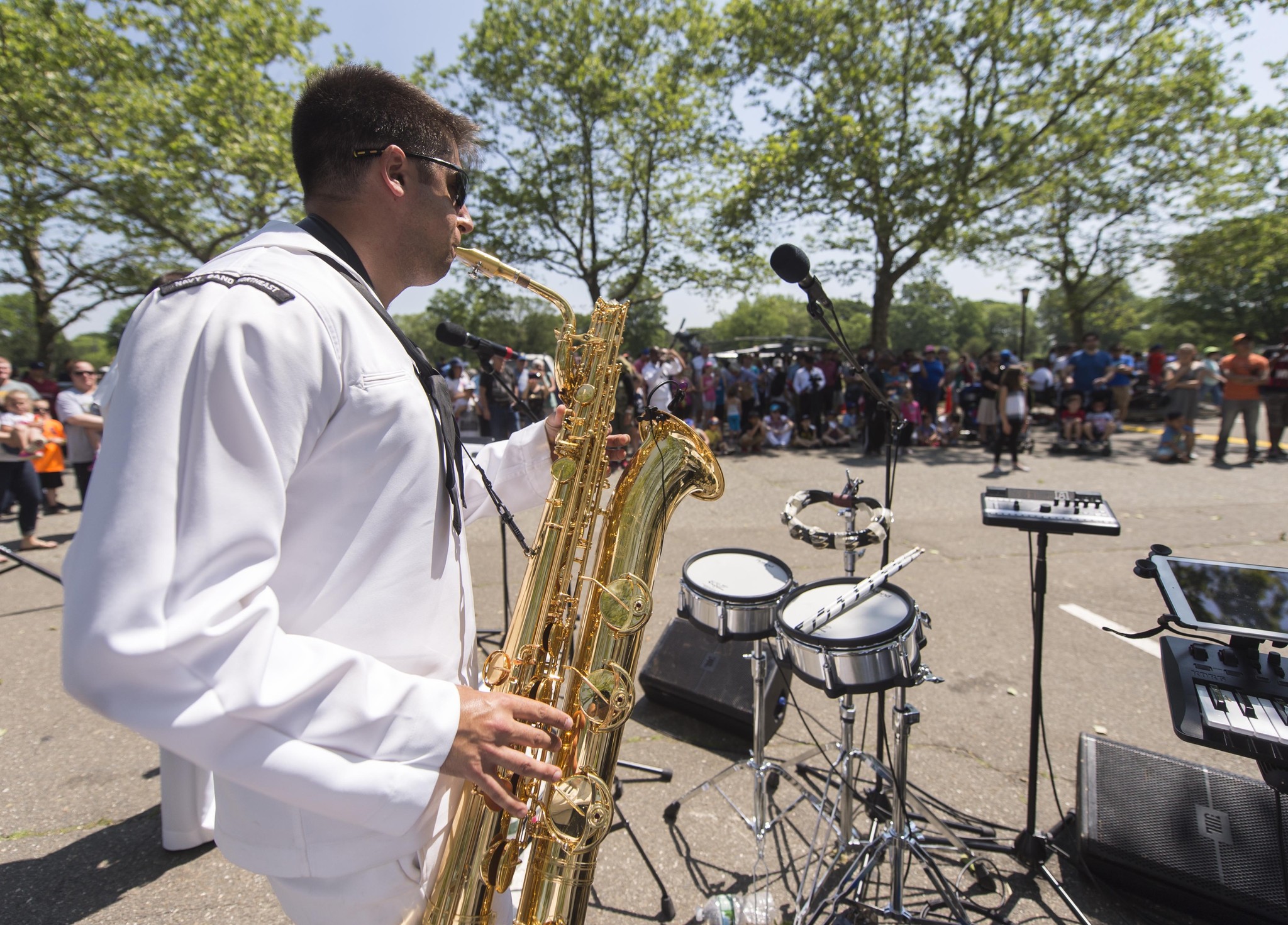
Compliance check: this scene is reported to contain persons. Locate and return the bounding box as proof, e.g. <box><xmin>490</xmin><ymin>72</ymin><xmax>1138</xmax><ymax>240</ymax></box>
<box><xmin>1191</xmin><ymin>345</ymin><xmax>1229</xmax><ymax>435</ymax></box>
<box><xmin>1163</xmin><ymin>343</ymin><xmax>1207</xmax><ymax>440</ymax></box>
<box><xmin>1257</xmin><ymin>339</ymin><xmax>1288</xmax><ymax>464</ymax></box>
<box><xmin>0</xmin><ymin>357</ymin><xmax>59</xmax><ymax>552</ymax></box>
<box><xmin>0</xmin><ymin>390</ymin><xmax>48</xmax><ymax>454</ymax></box>
<box><xmin>54</xmin><ymin>360</ymin><xmax>106</xmax><ymax>510</ymax></box>
<box><xmin>85</xmin><ymin>364</ymin><xmax>115</xmax><ymax>471</ymax></box>
<box><xmin>435</xmin><ymin>324</ymin><xmax>1169</xmax><ymax>466</ymax></box>
<box><xmin>58</xmin><ymin>65</ymin><xmax>590</xmax><ymax>923</ymax></box>
<box><xmin>1213</xmin><ymin>333</ymin><xmax>1271</xmax><ymax>465</ymax></box>
<box><xmin>1154</xmin><ymin>408</ymin><xmax>1196</xmax><ymax>464</ymax></box>
<box><xmin>27</xmin><ymin>399</ymin><xmax>71</xmax><ymax>517</ymax></box>
<box><xmin>20</xmin><ymin>360</ymin><xmax>63</xmax><ymax>419</ymax></box>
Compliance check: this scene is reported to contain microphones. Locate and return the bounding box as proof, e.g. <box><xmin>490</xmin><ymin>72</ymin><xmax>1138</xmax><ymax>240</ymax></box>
<box><xmin>435</xmin><ymin>322</ymin><xmax>525</xmax><ymax>364</ymax></box>
<box><xmin>769</xmin><ymin>245</ymin><xmax>836</xmax><ymax>314</ymax></box>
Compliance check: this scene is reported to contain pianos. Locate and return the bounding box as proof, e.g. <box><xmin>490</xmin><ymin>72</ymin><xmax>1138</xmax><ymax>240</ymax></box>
<box><xmin>1157</xmin><ymin>635</ymin><xmax>1288</xmax><ymax>900</ymax></box>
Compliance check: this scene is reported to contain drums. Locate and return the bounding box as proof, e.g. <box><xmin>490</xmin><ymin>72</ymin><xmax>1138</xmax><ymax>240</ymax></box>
<box><xmin>677</xmin><ymin>546</ymin><xmax>794</xmax><ymax>639</ymax></box>
<box><xmin>773</xmin><ymin>574</ymin><xmax>927</xmax><ymax>694</ymax></box>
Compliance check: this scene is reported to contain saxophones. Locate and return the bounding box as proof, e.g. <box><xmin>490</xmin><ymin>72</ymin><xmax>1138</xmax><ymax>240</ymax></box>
<box><xmin>391</xmin><ymin>230</ymin><xmax>726</xmax><ymax>925</ymax></box>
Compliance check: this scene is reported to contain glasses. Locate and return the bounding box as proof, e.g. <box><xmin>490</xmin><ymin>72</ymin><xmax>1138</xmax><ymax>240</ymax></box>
<box><xmin>354</xmin><ymin>149</ymin><xmax>468</xmax><ymax>208</ymax></box>
<box><xmin>71</xmin><ymin>371</ymin><xmax>94</xmax><ymax>376</ymax></box>
